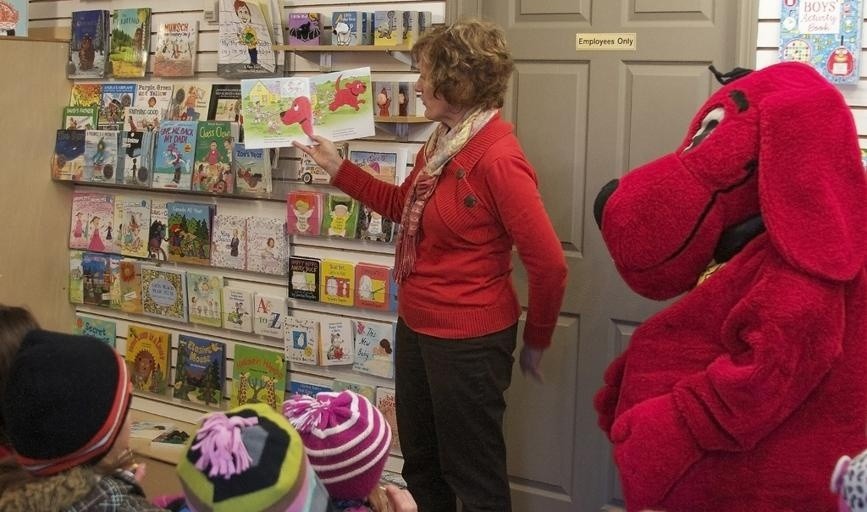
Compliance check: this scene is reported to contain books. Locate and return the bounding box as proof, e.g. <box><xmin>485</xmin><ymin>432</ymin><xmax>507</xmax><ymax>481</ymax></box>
<box><xmin>0</xmin><ymin>0</ymin><xmax>29</xmax><ymax>38</ymax></box>
<box><xmin>49</xmin><ymin>0</ymin><xmax>433</xmax><ymax>493</ymax></box>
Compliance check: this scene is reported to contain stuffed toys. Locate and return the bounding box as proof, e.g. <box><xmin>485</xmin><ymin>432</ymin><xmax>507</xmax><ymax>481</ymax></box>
<box><xmin>591</xmin><ymin>59</ymin><xmax>867</xmax><ymax>511</ymax></box>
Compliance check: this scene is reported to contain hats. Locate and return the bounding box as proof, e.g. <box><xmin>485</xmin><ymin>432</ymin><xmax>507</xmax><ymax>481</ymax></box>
<box><xmin>0</xmin><ymin>329</ymin><xmax>132</xmax><ymax>477</ymax></box>
<box><xmin>282</xmin><ymin>390</ymin><xmax>394</xmax><ymax>502</ymax></box>
<box><xmin>176</xmin><ymin>402</ymin><xmax>330</xmax><ymax>512</ymax></box>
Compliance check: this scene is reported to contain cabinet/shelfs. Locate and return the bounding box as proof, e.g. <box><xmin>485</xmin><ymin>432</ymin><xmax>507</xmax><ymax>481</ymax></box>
<box><xmin>271</xmin><ymin>45</ymin><xmax>436</xmax><ymax>124</ymax></box>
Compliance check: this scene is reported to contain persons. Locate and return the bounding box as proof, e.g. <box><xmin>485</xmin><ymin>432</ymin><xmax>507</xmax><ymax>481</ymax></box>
<box><xmin>0</xmin><ymin>300</ymin><xmax>42</xmax><ymax>392</ymax></box>
<box><xmin>290</xmin><ymin>19</ymin><xmax>570</xmax><ymax>512</ymax></box>
<box><xmin>0</xmin><ymin>327</ymin><xmax>171</xmax><ymax>512</ymax></box>
<box><xmin>150</xmin><ymin>401</ymin><xmax>330</xmax><ymax>510</ymax></box>
<box><xmin>282</xmin><ymin>388</ymin><xmax>418</xmax><ymax>512</ymax></box>
